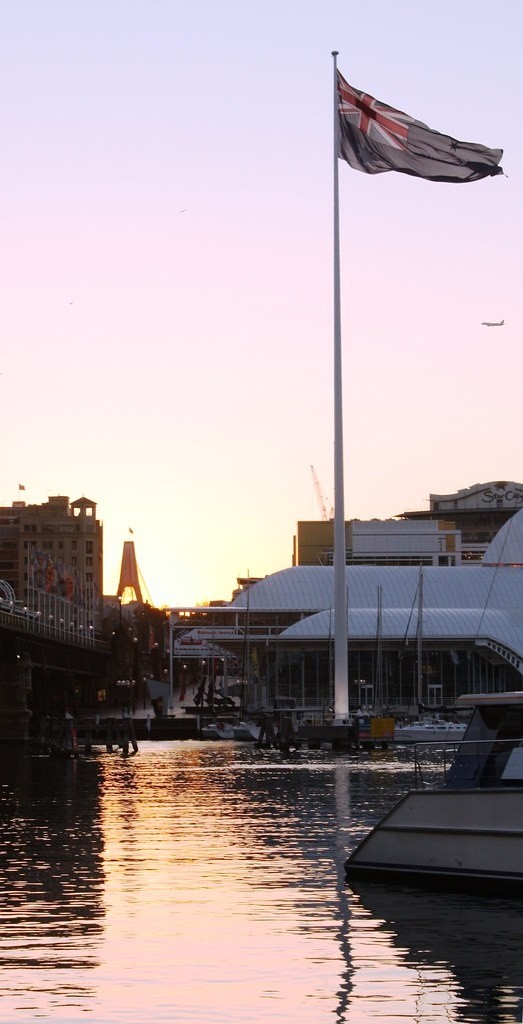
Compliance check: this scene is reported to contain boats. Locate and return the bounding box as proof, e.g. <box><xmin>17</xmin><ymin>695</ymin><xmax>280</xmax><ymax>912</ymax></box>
<box><xmin>344</xmin><ymin>692</ymin><xmax>523</xmax><ymax>892</ymax></box>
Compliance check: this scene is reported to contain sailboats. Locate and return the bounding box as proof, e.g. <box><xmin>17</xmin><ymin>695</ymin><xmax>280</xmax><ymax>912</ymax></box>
<box><xmin>200</xmin><ymin>562</ymin><xmax>469</xmax><ymax>743</ymax></box>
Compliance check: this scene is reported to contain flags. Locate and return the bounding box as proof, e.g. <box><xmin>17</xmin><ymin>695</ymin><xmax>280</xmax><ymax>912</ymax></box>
<box><xmin>19</xmin><ymin>484</ymin><xmax>25</xmax><ymax>490</ymax></box>
<box><xmin>336</xmin><ymin>69</ymin><xmax>504</xmax><ymax>183</ymax></box>
<box><xmin>26</xmin><ymin>541</ymin><xmax>100</xmax><ymax>614</ymax></box>
